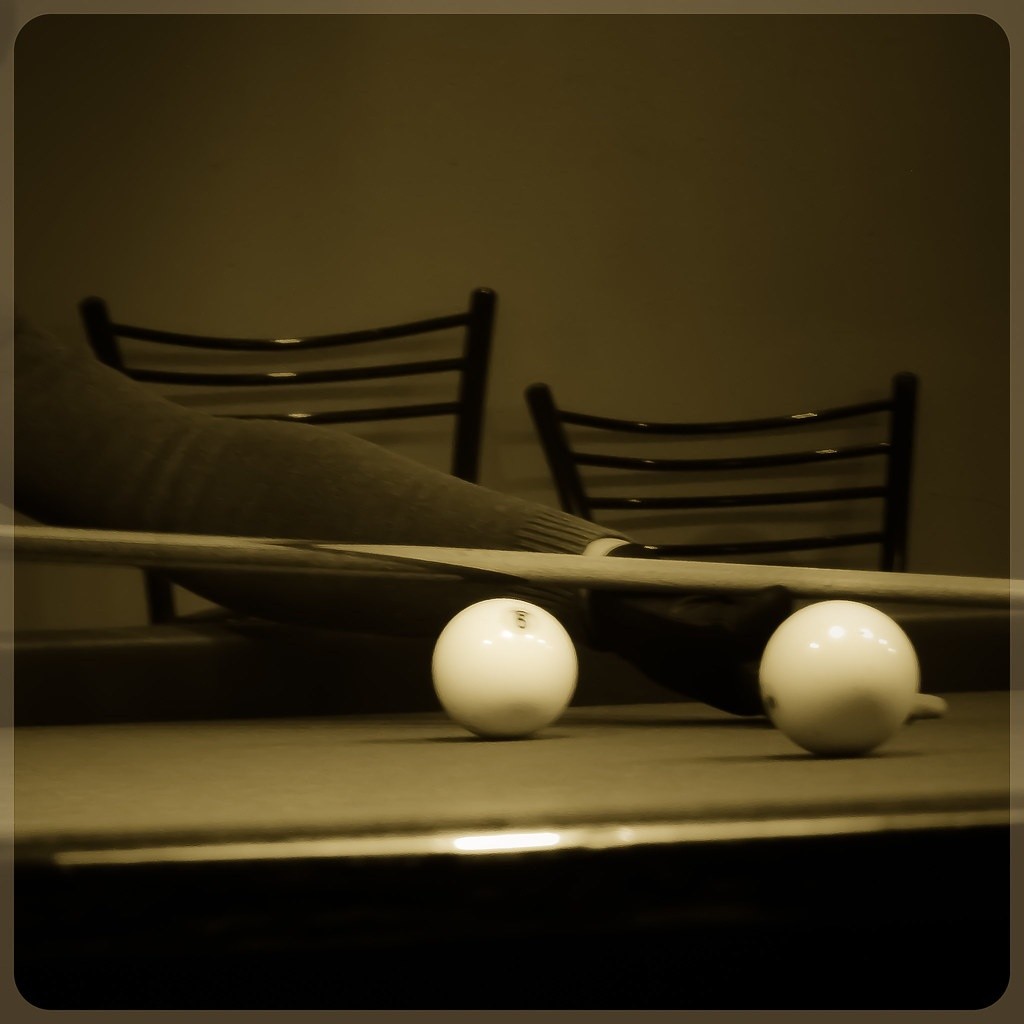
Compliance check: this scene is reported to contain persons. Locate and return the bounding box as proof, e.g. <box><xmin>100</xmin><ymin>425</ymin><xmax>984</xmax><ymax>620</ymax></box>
<box><xmin>15</xmin><ymin>312</ymin><xmax>948</xmax><ymax>722</ymax></box>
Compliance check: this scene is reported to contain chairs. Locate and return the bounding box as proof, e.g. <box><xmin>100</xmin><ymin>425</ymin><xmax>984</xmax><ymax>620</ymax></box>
<box><xmin>522</xmin><ymin>366</ymin><xmax>923</xmax><ymax>594</ymax></box>
<box><xmin>72</xmin><ymin>288</ymin><xmax>504</xmax><ymax>617</ymax></box>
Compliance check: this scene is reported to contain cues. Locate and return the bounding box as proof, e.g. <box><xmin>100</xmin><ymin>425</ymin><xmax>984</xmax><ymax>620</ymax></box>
<box><xmin>0</xmin><ymin>525</ymin><xmax>1023</xmax><ymax>608</ymax></box>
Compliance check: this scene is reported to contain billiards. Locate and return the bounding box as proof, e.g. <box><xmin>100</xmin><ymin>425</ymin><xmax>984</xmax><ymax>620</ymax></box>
<box><xmin>431</xmin><ymin>597</ymin><xmax>578</xmax><ymax>739</ymax></box>
<box><xmin>758</xmin><ymin>599</ymin><xmax>920</xmax><ymax>758</ymax></box>
